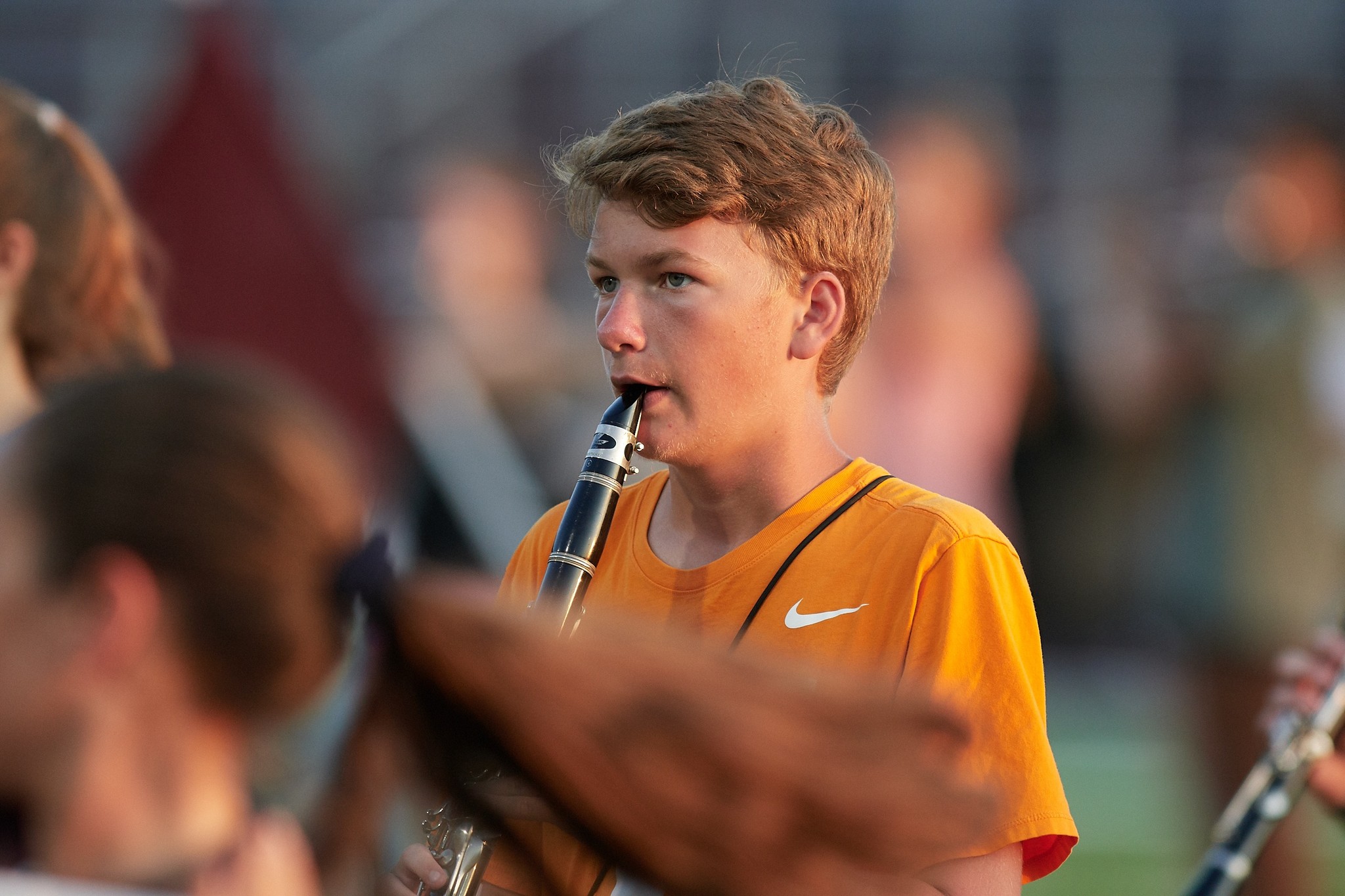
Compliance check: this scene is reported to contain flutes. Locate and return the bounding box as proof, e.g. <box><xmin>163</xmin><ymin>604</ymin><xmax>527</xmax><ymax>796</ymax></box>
<box><xmin>411</xmin><ymin>382</ymin><xmax>651</xmax><ymax>896</ymax></box>
<box><xmin>1180</xmin><ymin>658</ymin><xmax>1345</xmax><ymax>896</ymax></box>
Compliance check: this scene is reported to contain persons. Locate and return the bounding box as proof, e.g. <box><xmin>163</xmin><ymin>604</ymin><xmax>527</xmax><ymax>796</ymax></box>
<box><xmin>378</xmin><ymin>72</ymin><xmax>1078</xmax><ymax>895</ymax></box>
<box><xmin>1</xmin><ymin>352</ymin><xmax>1000</xmax><ymax>896</ymax></box>
<box><xmin>1</xmin><ymin>79</ymin><xmax>175</xmax><ymax>432</ymax></box>
<box><xmin>1253</xmin><ymin>620</ymin><xmax>1345</xmax><ymax>821</ymax></box>
